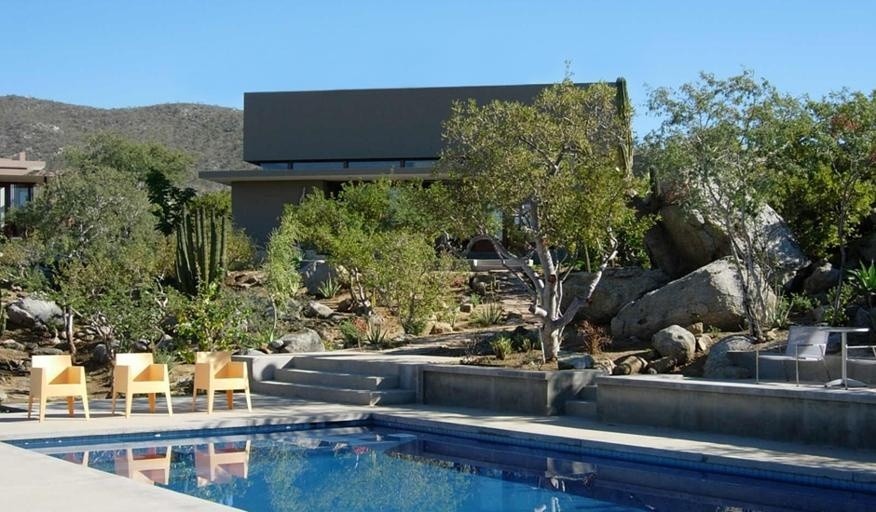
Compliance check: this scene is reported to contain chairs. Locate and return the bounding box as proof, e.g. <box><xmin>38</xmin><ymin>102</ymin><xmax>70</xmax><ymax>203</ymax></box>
<box><xmin>112</xmin><ymin>353</ymin><xmax>173</xmax><ymax>416</ymax></box>
<box><xmin>192</xmin><ymin>351</ymin><xmax>253</xmax><ymax>414</ymax></box>
<box><xmin>843</xmin><ymin>344</ymin><xmax>875</xmax><ymax>388</ymax></box>
<box><xmin>29</xmin><ymin>355</ymin><xmax>90</xmax><ymax>422</ymax></box>
<box><xmin>114</xmin><ymin>448</ymin><xmax>171</xmax><ymax>486</ymax></box>
<box><xmin>69</xmin><ymin>452</ymin><xmax>89</xmax><ymax>467</ymax></box>
<box><xmin>754</xmin><ymin>327</ymin><xmax>829</xmax><ymax>387</ymax></box>
<box><xmin>195</xmin><ymin>441</ymin><xmax>251</xmax><ymax>487</ymax></box>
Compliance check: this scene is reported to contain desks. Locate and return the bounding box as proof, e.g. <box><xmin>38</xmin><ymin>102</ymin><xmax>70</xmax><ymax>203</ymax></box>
<box><xmin>828</xmin><ymin>328</ymin><xmax>871</xmax><ymax>388</ymax></box>
